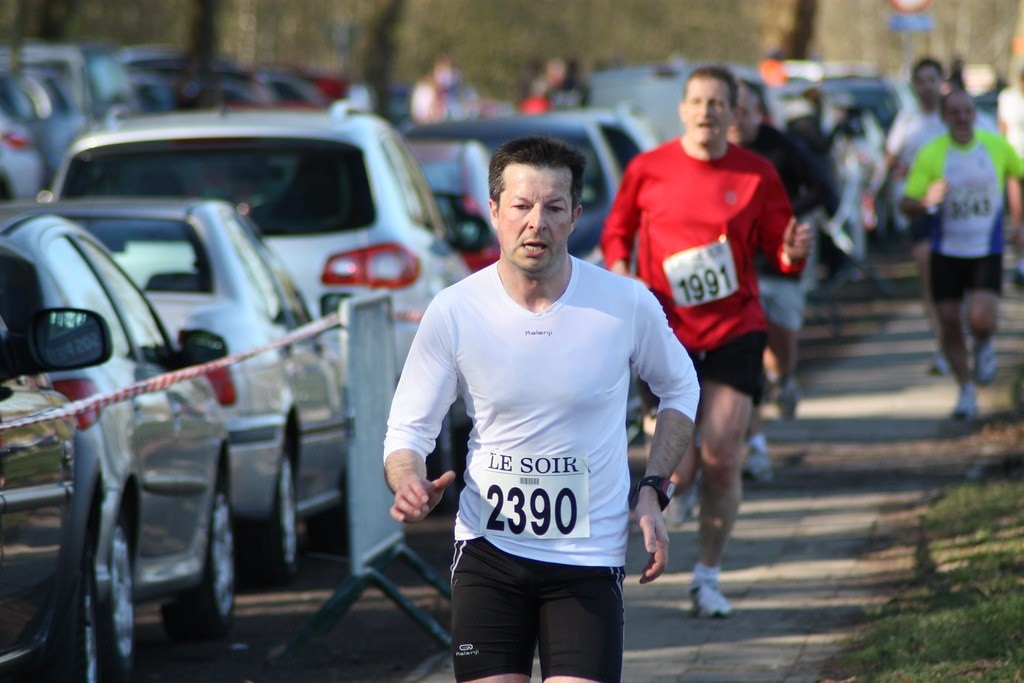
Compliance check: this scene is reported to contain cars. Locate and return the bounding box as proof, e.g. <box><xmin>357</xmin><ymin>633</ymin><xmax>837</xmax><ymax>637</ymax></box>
<box><xmin>0</xmin><ymin>194</ymin><xmax>346</xmax><ymax>584</ymax></box>
<box><xmin>1</xmin><ymin>303</ymin><xmax>114</xmax><ymax>682</ymax></box>
<box><xmin>0</xmin><ymin>41</ymin><xmax>1016</xmax><ymax>283</ymax></box>
<box><xmin>0</xmin><ymin>211</ymin><xmax>239</xmax><ymax>675</ymax></box>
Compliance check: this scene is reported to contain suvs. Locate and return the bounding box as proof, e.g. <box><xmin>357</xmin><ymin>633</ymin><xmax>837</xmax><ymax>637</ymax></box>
<box><xmin>43</xmin><ymin>98</ymin><xmax>493</xmax><ymax>512</ymax></box>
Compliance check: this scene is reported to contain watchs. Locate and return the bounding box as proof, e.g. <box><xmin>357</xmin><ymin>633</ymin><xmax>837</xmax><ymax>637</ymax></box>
<box><xmin>629</xmin><ymin>476</ymin><xmax>677</xmax><ymax>513</ymax></box>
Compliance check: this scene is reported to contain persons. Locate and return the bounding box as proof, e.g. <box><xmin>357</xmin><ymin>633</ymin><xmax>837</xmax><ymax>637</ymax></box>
<box><xmin>901</xmin><ymin>88</ymin><xmax>1024</xmax><ymax>422</ymax></box>
<box><xmin>408</xmin><ymin>52</ymin><xmax>586</xmax><ymax>125</ymax></box>
<box><xmin>383</xmin><ymin>136</ymin><xmax>699</xmax><ymax>683</ymax></box>
<box><xmin>600</xmin><ymin>65</ymin><xmax>814</xmax><ymax>617</ymax></box>
<box><xmin>725</xmin><ymin>58</ymin><xmax>1024</xmax><ymax>486</ymax></box>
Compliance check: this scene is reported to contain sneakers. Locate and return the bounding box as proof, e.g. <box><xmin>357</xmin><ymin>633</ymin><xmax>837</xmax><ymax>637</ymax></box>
<box><xmin>667</xmin><ymin>484</ymin><xmax>700</xmax><ymax>524</ymax></box>
<box><xmin>750</xmin><ymin>442</ymin><xmax>772</xmax><ymax>479</ymax></box>
<box><xmin>973</xmin><ymin>343</ymin><xmax>997</xmax><ymax>385</ymax></box>
<box><xmin>951</xmin><ymin>390</ymin><xmax>979</xmax><ymax>421</ymax></box>
<box><xmin>930</xmin><ymin>355</ymin><xmax>953</xmax><ymax>374</ymax></box>
<box><xmin>690</xmin><ymin>581</ymin><xmax>734</xmax><ymax>617</ymax></box>
